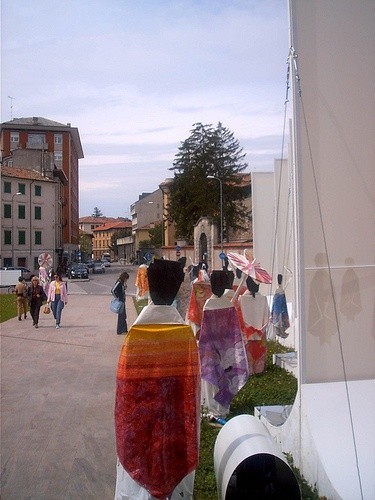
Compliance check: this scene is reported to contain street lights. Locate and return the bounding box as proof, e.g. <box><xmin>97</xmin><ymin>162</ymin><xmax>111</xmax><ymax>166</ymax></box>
<box><xmin>11</xmin><ymin>191</ymin><xmax>22</xmax><ymax>267</ymax></box>
<box><xmin>207</xmin><ymin>175</ymin><xmax>225</xmax><ymax>269</ymax></box>
<box><xmin>148</xmin><ymin>201</ymin><xmax>165</xmax><ymax>247</ymax></box>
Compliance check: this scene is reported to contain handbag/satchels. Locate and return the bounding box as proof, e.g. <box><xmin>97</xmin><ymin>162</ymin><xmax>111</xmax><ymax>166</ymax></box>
<box><xmin>43</xmin><ymin>303</ymin><xmax>50</xmax><ymax>314</ymax></box>
<box><xmin>110</xmin><ymin>298</ymin><xmax>123</xmax><ymax>314</ymax></box>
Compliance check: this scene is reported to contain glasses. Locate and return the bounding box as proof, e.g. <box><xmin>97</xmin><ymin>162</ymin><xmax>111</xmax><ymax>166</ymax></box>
<box><xmin>54</xmin><ymin>277</ymin><xmax>58</xmax><ymax>278</ymax></box>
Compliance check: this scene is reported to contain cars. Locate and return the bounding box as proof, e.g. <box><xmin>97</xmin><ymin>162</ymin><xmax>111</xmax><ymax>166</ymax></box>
<box><xmin>65</xmin><ymin>252</ymin><xmax>111</xmax><ymax>280</ymax></box>
<box><xmin>93</xmin><ymin>262</ymin><xmax>106</xmax><ymax>274</ymax></box>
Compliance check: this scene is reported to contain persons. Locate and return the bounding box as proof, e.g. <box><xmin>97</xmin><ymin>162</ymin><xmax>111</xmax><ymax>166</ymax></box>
<box><xmin>28</xmin><ymin>276</ymin><xmax>48</xmax><ymax>328</ymax></box>
<box><xmin>15</xmin><ymin>275</ymin><xmax>28</xmax><ymax>320</ymax></box>
<box><xmin>47</xmin><ymin>273</ymin><xmax>67</xmax><ymax>329</ymax></box>
<box><xmin>111</xmin><ymin>272</ymin><xmax>129</xmax><ymax>334</ymax></box>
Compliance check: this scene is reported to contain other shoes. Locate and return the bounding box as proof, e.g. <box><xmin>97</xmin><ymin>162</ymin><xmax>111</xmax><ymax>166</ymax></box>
<box><xmin>35</xmin><ymin>323</ymin><xmax>37</xmax><ymax>327</ymax></box>
<box><xmin>19</xmin><ymin>317</ymin><xmax>21</xmax><ymax>320</ymax></box>
<box><xmin>117</xmin><ymin>331</ymin><xmax>128</xmax><ymax>335</ymax></box>
<box><xmin>55</xmin><ymin>325</ymin><xmax>59</xmax><ymax>328</ymax></box>
<box><xmin>25</xmin><ymin>316</ymin><xmax>27</xmax><ymax>319</ymax></box>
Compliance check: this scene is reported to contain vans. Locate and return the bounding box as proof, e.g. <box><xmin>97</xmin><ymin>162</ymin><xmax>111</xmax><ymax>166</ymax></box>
<box><xmin>3</xmin><ymin>267</ymin><xmax>35</xmax><ymax>282</ymax></box>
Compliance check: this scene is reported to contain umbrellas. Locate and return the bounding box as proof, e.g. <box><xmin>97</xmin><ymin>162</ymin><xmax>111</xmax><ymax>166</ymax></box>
<box><xmin>227</xmin><ymin>250</ymin><xmax>272</xmax><ymax>304</ymax></box>
